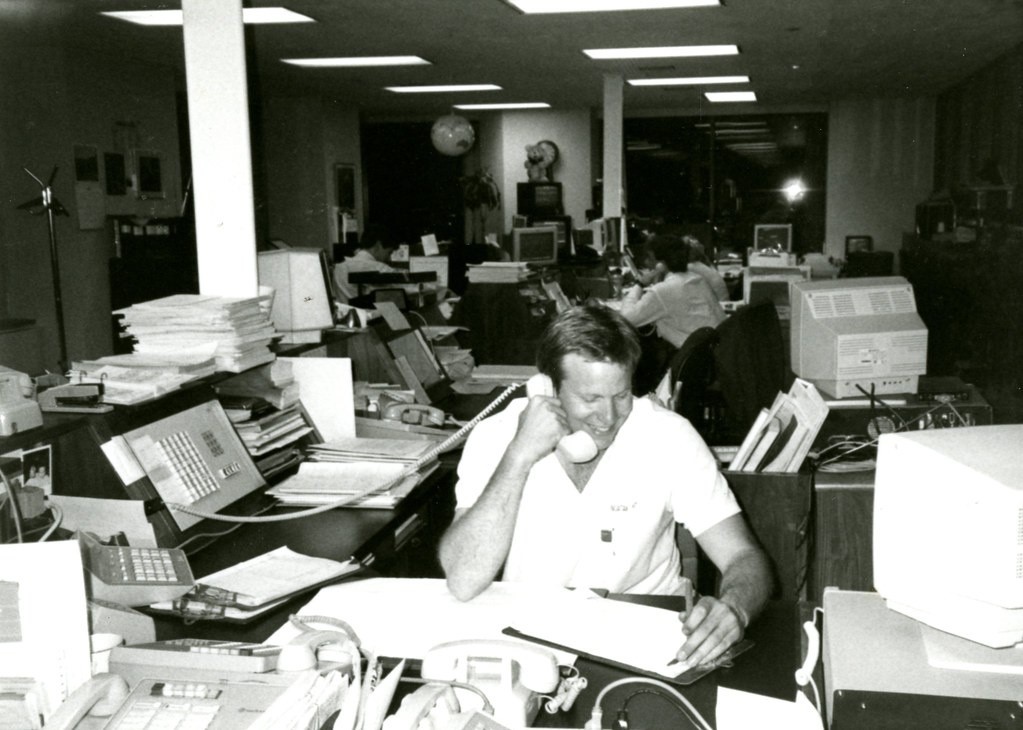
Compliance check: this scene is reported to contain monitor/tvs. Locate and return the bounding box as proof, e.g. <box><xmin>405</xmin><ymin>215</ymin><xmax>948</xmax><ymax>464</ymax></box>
<box><xmin>743</xmin><ymin>223</ymin><xmax>923</xmax><ymax>398</ymax></box>
<box><xmin>870</xmin><ymin>425</ymin><xmax>1023</xmax><ymax>649</ymax></box>
<box><xmin>513</xmin><ymin>182</ymin><xmax>620</xmax><ymax>272</ymax></box>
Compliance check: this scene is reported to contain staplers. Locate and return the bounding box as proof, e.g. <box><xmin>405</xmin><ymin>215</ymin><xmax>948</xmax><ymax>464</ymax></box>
<box><xmin>37</xmin><ymin>383</ymin><xmax>114</xmax><ymax>414</ymax></box>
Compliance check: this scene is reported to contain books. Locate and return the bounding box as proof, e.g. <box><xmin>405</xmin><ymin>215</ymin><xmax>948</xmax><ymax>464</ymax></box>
<box><xmin>729</xmin><ymin>377</ymin><xmax>829</xmax><ymax>471</ymax></box>
<box><xmin>216</xmin><ymin>396</ymin><xmax>312</xmax><ymax>457</ymax></box>
<box><xmin>466</xmin><ymin>263</ymin><xmax>534</xmax><ymax>284</ymax></box>
<box><xmin>112</xmin><ymin>295</ymin><xmax>279</xmax><ymax>374</ymax></box>
<box><xmin>77</xmin><ymin>355</ymin><xmax>216</xmax><ymax>406</ymax></box>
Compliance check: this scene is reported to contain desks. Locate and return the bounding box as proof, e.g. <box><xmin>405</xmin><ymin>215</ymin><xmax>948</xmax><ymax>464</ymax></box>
<box><xmin>822</xmin><ymin>383</ymin><xmax>994</xmax><ymax>456</ymax></box>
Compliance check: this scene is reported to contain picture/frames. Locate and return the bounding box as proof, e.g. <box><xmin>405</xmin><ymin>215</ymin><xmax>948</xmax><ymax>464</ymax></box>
<box><xmin>333</xmin><ymin>163</ymin><xmax>358</xmax><ymax>215</ymax></box>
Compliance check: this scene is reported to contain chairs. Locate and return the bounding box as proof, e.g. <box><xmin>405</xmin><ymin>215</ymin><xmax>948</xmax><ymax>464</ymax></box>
<box><xmin>696</xmin><ymin>471</ymin><xmax>812</xmax><ymax>602</ymax></box>
<box><xmin>678</xmin><ymin>298</ymin><xmax>776</xmax><ymax>442</ymax></box>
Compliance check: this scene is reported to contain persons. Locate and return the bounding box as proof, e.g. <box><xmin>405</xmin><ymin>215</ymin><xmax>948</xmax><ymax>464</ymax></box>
<box><xmin>439</xmin><ymin>302</ymin><xmax>777</xmax><ymax>670</ymax></box>
<box><xmin>334</xmin><ymin>224</ymin><xmax>430</xmax><ymax>304</ymax></box>
<box><xmin>619</xmin><ymin>235</ymin><xmax>728</xmax><ymax>393</ymax></box>
<box><xmin>26</xmin><ymin>464</ymin><xmax>50</xmax><ymax>494</ymax></box>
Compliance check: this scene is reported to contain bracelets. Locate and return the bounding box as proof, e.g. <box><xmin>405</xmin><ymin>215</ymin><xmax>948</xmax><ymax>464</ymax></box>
<box><xmin>634</xmin><ymin>280</ymin><xmax>645</xmax><ymax>289</ymax></box>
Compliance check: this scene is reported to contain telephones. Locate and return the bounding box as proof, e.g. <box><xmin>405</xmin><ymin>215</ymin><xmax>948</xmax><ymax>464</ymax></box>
<box><xmin>35</xmin><ymin>672</ymin><xmax>288</xmax><ymax>730</ymax></box>
<box><xmin>70</xmin><ymin>530</ymin><xmax>196</xmax><ymax>608</ymax></box>
<box><xmin>381</xmin><ymin>680</ymin><xmax>511</xmax><ymax>730</ymax></box>
<box><xmin>526</xmin><ymin>372</ymin><xmax>599</xmax><ymax>464</ymax></box>
<box><xmin>107</xmin><ymin>614</ymin><xmax>370</xmax><ymax>693</ymax></box>
<box><xmin>421</xmin><ymin>638</ymin><xmax>561</xmax><ymax>729</ymax></box>
<box><xmin>355</xmin><ymin>403</ymin><xmax>454</xmax><ymax>442</ymax></box>
<box><xmin>646</xmin><ymin>262</ymin><xmax>665</xmax><ymax>279</ymax></box>
<box><xmin>0</xmin><ymin>363</ymin><xmax>46</xmax><ymax>439</ymax></box>
